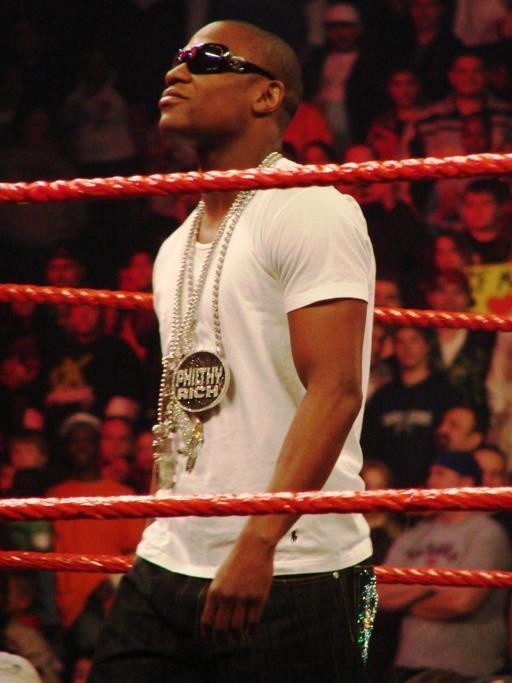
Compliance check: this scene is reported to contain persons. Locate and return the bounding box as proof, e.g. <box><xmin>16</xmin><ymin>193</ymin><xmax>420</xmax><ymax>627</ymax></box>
<box><xmin>0</xmin><ymin>3</ymin><xmax>511</xmax><ymax>681</ymax></box>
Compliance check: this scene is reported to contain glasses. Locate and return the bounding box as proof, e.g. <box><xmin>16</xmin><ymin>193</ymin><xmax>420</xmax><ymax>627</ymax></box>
<box><xmin>170</xmin><ymin>44</ymin><xmax>278</xmax><ymax>80</ymax></box>
